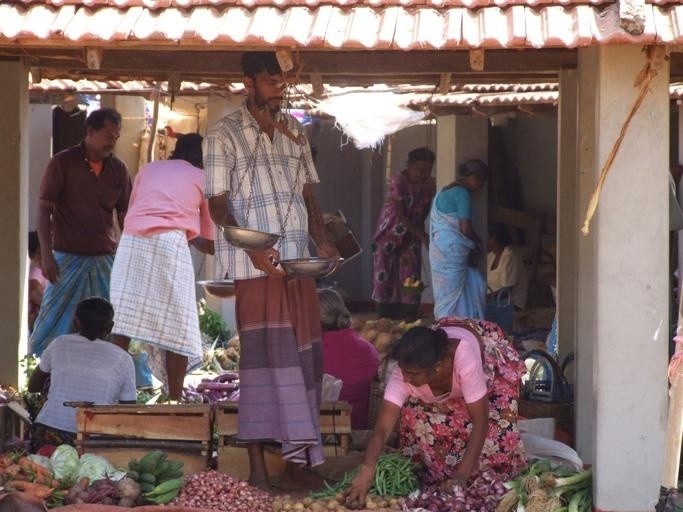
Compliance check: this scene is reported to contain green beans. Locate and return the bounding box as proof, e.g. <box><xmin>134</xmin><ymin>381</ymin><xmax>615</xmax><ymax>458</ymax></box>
<box><xmin>310</xmin><ymin>451</ymin><xmax>419</xmax><ymax>498</ymax></box>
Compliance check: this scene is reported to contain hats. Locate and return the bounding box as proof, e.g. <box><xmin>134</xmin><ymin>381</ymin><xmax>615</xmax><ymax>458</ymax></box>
<box><xmin>409</xmin><ymin>147</ymin><xmax>438</xmax><ymax>165</ymax></box>
<box><xmin>240</xmin><ymin>51</ymin><xmax>288</xmax><ymax>68</ymax></box>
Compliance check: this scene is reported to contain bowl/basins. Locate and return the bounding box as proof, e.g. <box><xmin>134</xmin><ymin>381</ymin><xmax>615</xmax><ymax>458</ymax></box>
<box><xmin>278</xmin><ymin>256</ymin><xmax>344</xmax><ymax>280</ymax></box>
<box><xmin>217</xmin><ymin>224</ymin><xmax>284</xmax><ymax>251</ymax></box>
<box><xmin>195</xmin><ymin>279</ymin><xmax>235</xmax><ymax>298</ymax></box>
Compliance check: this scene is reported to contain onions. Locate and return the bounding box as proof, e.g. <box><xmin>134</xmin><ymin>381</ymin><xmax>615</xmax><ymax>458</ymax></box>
<box><xmin>404</xmin><ymin>483</ymin><xmax>500</xmax><ymax>512</ymax></box>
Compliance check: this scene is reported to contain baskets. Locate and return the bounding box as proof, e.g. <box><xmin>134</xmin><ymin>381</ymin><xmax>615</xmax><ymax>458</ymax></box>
<box><xmin>515</xmin><ymin>347</ymin><xmax>576</xmax><ymax>436</ymax></box>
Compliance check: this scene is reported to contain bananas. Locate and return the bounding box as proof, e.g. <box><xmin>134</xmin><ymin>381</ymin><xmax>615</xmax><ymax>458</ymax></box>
<box><xmin>127</xmin><ymin>450</ymin><xmax>185</xmax><ymax>504</ymax></box>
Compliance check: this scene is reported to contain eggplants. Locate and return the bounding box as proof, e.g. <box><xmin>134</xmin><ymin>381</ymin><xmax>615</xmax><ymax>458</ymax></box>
<box><xmin>160</xmin><ymin>373</ymin><xmax>241</xmax><ymax>403</ymax></box>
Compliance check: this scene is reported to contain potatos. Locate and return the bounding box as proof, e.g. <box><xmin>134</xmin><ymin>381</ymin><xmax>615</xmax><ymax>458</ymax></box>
<box><xmin>272</xmin><ymin>494</ymin><xmax>402</xmax><ymax>512</ymax></box>
<box><xmin>116</xmin><ymin>477</ymin><xmax>139</xmax><ymax>508</ymax></box>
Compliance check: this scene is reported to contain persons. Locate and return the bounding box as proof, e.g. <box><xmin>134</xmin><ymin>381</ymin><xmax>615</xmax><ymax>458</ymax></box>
<box><xmin>27</xmin><ymin>294</ymin><xmax>136</xmax><ymax>448</ymax></box>
<box><xmin>342</xmin><ymin>315</ymin><xmax>527</xmax><ymax>509</ymax></box>
<box><xmin>428</xmin><ymin>157</ymin><xmax>491</xmax><ymax>321</ymax></box>
<box><xmin>200</xmin><ymin>49</ymin><xmax>345</xmax><ymax>493</ymax></box>
<box><xmin>316</xmin><ymin>285</ymin><xmax>382</xmax><ymax>431</ymax></box>
<box><xmin>108</xmin><ymin>131</ymin><xmax>217</xmax><ymax>401</ymax></box>
<box><xmin>27</xmin><ymin>106</ymin><xmax>157</xmax><ymax>399</ymax></box>
<box><xmin>366</xmin><ymin>147</ymin><xmax>436</xmax><ymax>322</ymax></box>
<box><xmin>28</xmin><ymin>227</ymin><xmax>46</xmax><ymax>333</ymax></box>
<box><xmin>484</xmin><ymin>225</ymin><xmax>530</xmax><ymax>332</ymax></box>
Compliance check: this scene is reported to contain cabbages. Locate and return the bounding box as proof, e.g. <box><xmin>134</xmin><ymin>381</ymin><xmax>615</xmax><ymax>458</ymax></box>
<box><xmin>26</xmin><ymin>444</ymin><xmax>126</xmax><ymax>485</ymax></box>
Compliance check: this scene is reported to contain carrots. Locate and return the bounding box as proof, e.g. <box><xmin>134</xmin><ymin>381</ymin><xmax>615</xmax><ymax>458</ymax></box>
<box><xmin>0</xmin><ymin>450</ymin><xmax>73</xmax><ymax>506</ymax></box>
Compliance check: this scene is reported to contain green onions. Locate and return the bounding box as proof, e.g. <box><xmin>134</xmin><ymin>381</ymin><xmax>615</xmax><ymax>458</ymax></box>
<box><xmin>496</xmin><ymin>458</ymin><xmax>594</xmax><ymax>512</ymax></box>
